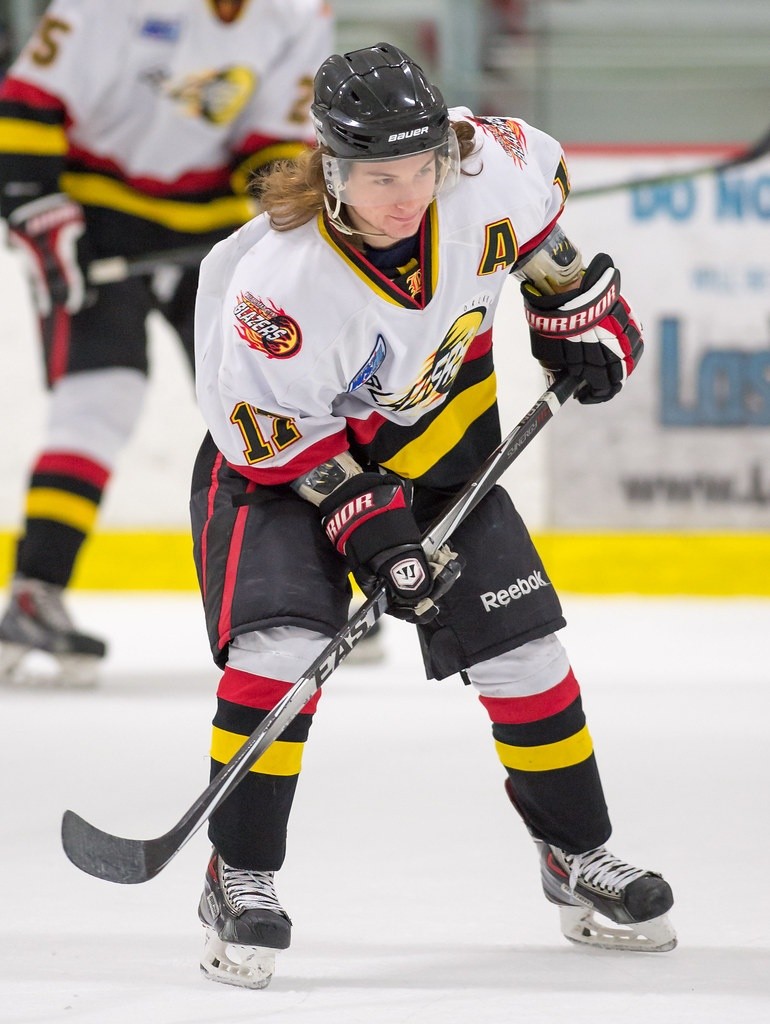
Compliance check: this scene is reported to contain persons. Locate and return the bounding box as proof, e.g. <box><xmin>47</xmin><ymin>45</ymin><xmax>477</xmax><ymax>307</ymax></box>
<box><xmin>0</xmin><ymin>4</ymin><xmax>383</xmax><ymax>692</ymax></box>
<box><xmin>199</xmin><ymin>43</ymin><xmax>678</xmax><ymax>991</ymax></box>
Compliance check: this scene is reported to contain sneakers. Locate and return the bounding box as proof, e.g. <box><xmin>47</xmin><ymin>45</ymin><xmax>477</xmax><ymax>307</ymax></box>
<box><xmin>352</xmin><ymin>621</ymin><xmax>386</xmax><ymax>663</ymax></box>
<box><xmin>196</xmin><ymin>845</ymin><xmax>292</xmax><ymax>990</ymax></box>
<box><xmin>0</xmin><ymin>541</ymin><xmax>106</xmax><ymax>687</ymax></box>
<box><xmin>505</xmin><ymin>776</ymin><xmax>677</xmax><ymax>952</ymax></box>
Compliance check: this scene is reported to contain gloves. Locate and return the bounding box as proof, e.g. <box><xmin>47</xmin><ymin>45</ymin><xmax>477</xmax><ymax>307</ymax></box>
<box><xmin>521</xmin><ymin>253</ymin><xmax>644</xmax><ymax>404</ymax></box>
<box><xmin>318</xmin><ymin>472</ymin><xmax>462</xmax><ymax>625</ymax></box>
<box><xmin>11</xmin><ymin>200</ymin><xmax>102</xmax><ymax>318</ymax></box>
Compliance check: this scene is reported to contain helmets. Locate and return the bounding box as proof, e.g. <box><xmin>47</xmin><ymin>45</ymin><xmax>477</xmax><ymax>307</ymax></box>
<box><xmin>310</xmin><ymin>42</ymin><xmax>460</xmax><ymax>207</ymax></box>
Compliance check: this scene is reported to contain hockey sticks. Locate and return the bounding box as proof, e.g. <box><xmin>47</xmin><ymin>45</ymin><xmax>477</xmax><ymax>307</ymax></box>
<box><xmin>60</xmin><ymin>360</ymin><xmax>586</xmax><ymax>884</ymax></box>
<box><xmin>90</xmin><ymin>128</ymin><xmax>770</xmax><ymax>286</ymax></box>
